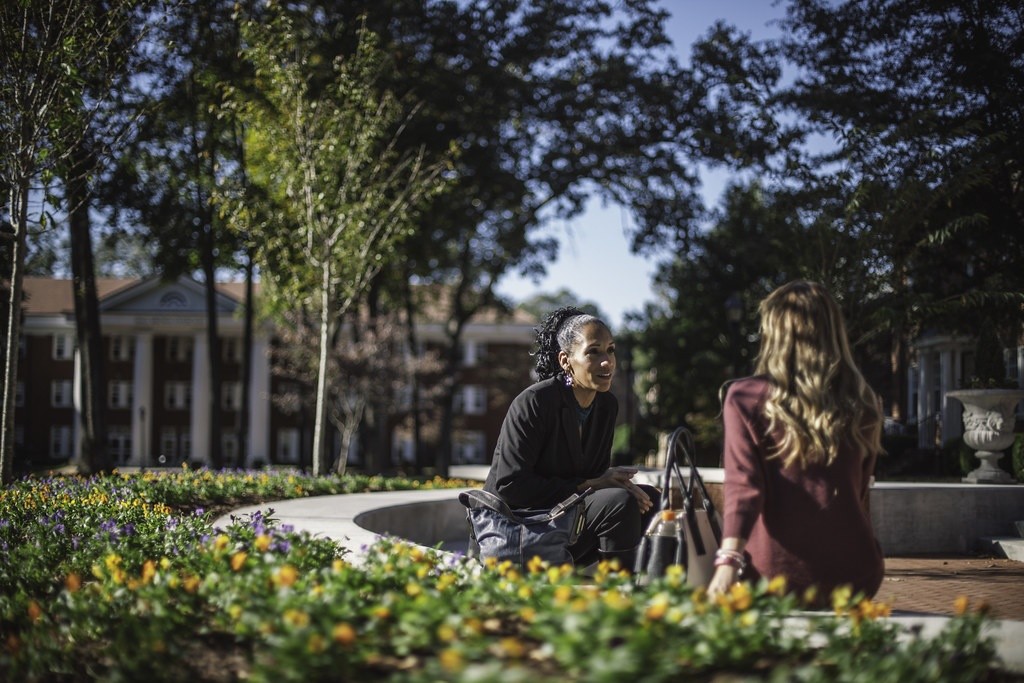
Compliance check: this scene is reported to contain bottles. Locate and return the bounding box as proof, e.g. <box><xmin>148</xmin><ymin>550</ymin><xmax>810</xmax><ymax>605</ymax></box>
<box><xmin>657</xmin><ymin>510</ymin><xmax>678</xmax><ymax>537</ymax></box>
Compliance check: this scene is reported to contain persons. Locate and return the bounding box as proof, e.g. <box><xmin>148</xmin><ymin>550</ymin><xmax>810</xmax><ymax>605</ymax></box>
<box><xmin>483</xmin><ymin>305</ymin><xmax>662</xmax><ymax>574</ymax></box>
<box><xmin>706</xmin><ymin>282</ymin><xmax>887</xmax><ymax>610</ymax></box>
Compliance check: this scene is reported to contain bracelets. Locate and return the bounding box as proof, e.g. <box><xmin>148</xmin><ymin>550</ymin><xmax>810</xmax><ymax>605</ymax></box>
<box><xmin>714</xmin><ymin>544</ymin><xmax>746</xmax><ymax>570</ymax></box>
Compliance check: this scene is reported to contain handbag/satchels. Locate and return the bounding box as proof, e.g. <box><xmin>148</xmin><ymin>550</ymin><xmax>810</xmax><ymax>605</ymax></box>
<box><xmin>635</xmin><ymin>428</ymin><xmax>724</xmax><ymax>594</ymax></box>
<box><xmin>459</xmin><ymin>488</ymin><xmax>593</xmax><ymax>574</ymax></box>
